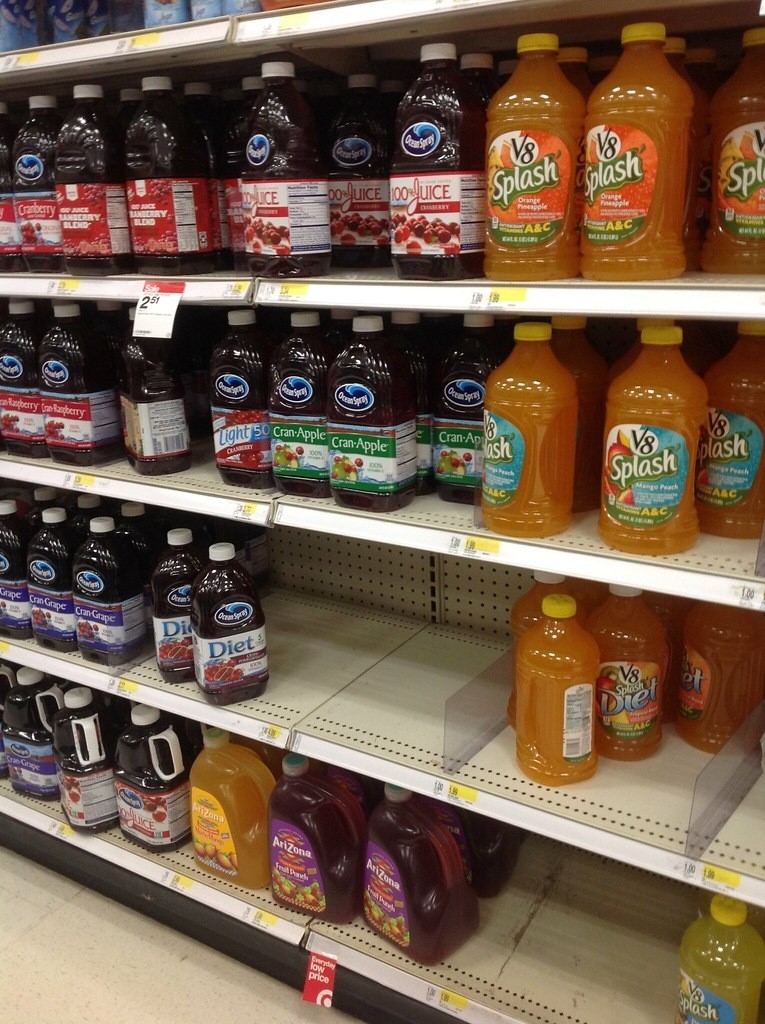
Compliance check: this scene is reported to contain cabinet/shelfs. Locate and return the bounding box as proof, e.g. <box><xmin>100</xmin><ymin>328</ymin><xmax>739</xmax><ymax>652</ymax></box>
<box><xmin>0</xmin><ymin>0</ymin><xmax>765</xmax><ymax>1024</ymax></box>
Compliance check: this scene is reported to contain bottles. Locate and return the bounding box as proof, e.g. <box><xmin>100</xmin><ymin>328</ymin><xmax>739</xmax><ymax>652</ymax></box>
<box><xmin>0</xmin><ymin>297</ymin><xmax>207</xmax><ymax>477</ymax></box>
<box><xmin>0</xmin><ymin>19</ymin><xmax>765</xmax><ymax>282</ymax></box>
<box><xmin>191</xmin><ymin>542</ymin><xmax>269</xmax><ymax>706</ymax></box>
<box><xmin>0</xmin><ymin>488</ymin><xmax>157</xmax><ymax>669</ymax></box>
<box><xmin>152</xmin><ymin>528</ymin><xmax>205</xmax><ymax>684</ymax></box>
<box><xmin>0</xmin><ymin>662</ymin><xmax>525</xmax><ymax>966</ymax></box>
<box><xmin>209</xmin><ymin>306</ymin><xmax>765</xmax><ymax>558</ymax></box>
<box><xmin>674</xmin><ymin>893</ymin><xmax>765</xmax><ymax>1024</ymax></box>
<box><xmin>506</xmin><ymin>571</ymin><xmax>765</xmax><ymax>787</ymax></box>
